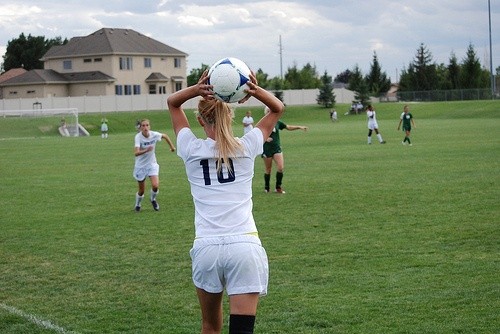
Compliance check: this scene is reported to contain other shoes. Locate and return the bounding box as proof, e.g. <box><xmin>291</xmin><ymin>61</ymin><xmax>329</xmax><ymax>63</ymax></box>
<box><xmin>402</xmin><ymin>141</ymin><xmax>405</xmax><ymax>146</ymax></box>
<box><xmin>380</xmin><ymin>141</ymin><xmax>387</xmax><ymax>144</ymax></box>
<box><xmin>265</xmin><ymin>189</ymin><xmax>271</xmax><ymax>193</ymax></box>
<box><xmin>275</xmin><ymin>189</ymin><xmax>286</xmax><ymax>194</ymax></box>
<box><xmin>135</xmin><ymin>201</ymin><xmax>141</xmax><ymax>211</ymax></box>
<box><xmin>408</xmin><ymin>143</ymin><xmax>413</xmax><ymax>147</ymax></box>
<box><xmin>149</xmin><ymin>197</ymin><xmax>159</xmax><ymax>210</ymax></box>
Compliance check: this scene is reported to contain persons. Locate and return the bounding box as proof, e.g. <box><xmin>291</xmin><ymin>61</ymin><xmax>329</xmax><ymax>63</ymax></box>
<box><xmin>242</xmin><ymin>111</ymin><xmax>254</xmax><ymax>135</ymax></box>
<box><xmin>366</xmin><ymin>105</ymin><xmax>386</xmax><ymax>145</ymax></box>
<box><xmin>330</xmin><ymin>101</ymin><xmax>363</xmax><ymax>121</ymax></box>
<box><xmin>100</xmin><ymin>117</ymin><xmax>109</xmax><ymax>138</ymax></box>
<box><xmin>167</xmin><ymin>69</ymin><xmax>284</xmax><ymax>334</ymax></box>
<box><xmin>134</xmin><ymin>119</ymin><xmax>176</xmax><ymax>211</ymax></box>
<box><xmin>398</xmin><ymin>106</ymin><xmax>416</xmax><ymax>146</ymax></box>
<box><xmin>261</xmin><ymin>107</ymin><xmax>307</xmax><ymax>194</ymax></box>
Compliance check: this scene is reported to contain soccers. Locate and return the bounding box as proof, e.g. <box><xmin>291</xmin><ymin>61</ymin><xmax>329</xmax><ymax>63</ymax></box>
<box><xmin>207</xmin><ymin>57</ymin><xmax>253</xmax><ymax>103</ymax></box>
